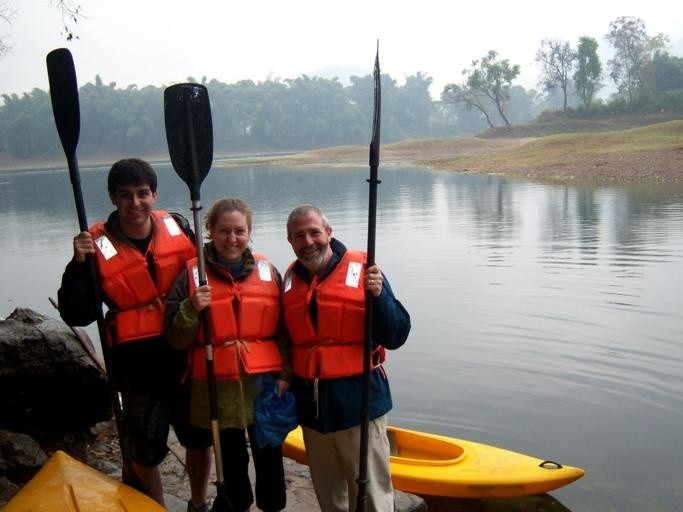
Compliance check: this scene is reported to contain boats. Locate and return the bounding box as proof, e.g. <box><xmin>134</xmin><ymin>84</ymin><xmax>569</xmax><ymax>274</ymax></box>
<box><xmin>0</xmin><ymin>449</ymin><xmax>167</xmax><ymax>512</ymax></box>
<box><xmin>282</xmin><ymin>425</ymin><xmax>586</xmax><ymax>499</ymax></box>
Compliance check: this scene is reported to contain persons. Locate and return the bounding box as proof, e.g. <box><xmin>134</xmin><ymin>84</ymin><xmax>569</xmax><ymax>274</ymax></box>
<box><xmin>57</xmin><ymin>157</ymin><xmax>213</xmax><ymax>511</ymax></box>
<box><xmin>280</xmin><ymin>205</ymin><xmax>409</xmax><ymax>512</ymax></box>
<box><xmin>161</xmin><ymin>198</ymin><xmax>294</xmax><ymax>512</ymax></box>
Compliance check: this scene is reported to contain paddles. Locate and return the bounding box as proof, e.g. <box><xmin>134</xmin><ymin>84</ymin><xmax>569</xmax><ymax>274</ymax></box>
<box><xmin>354</xmin><ymin>40</ymin><xmax>381</xmax><ymax>511</ymax></box>
<box><xmin>46</xmin><ymin>48</ymin><xmax>149</xmax><ymax>496</ymax></box>
<box><xmin>48</xmin><ymin>297</ymin><xmax>188</xmax><ymax>469</ymax></box>
<box><xmin>163</xmin><ymin>83</ymin><xmax>237</xmax><ymax>512</ymax></box>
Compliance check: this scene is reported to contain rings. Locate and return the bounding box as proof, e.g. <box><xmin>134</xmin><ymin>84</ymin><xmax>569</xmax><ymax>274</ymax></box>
<box><xmin>369</xmin><ymin>279</ymin><xmax>374</xmax><ymax>284</ymax></box>
